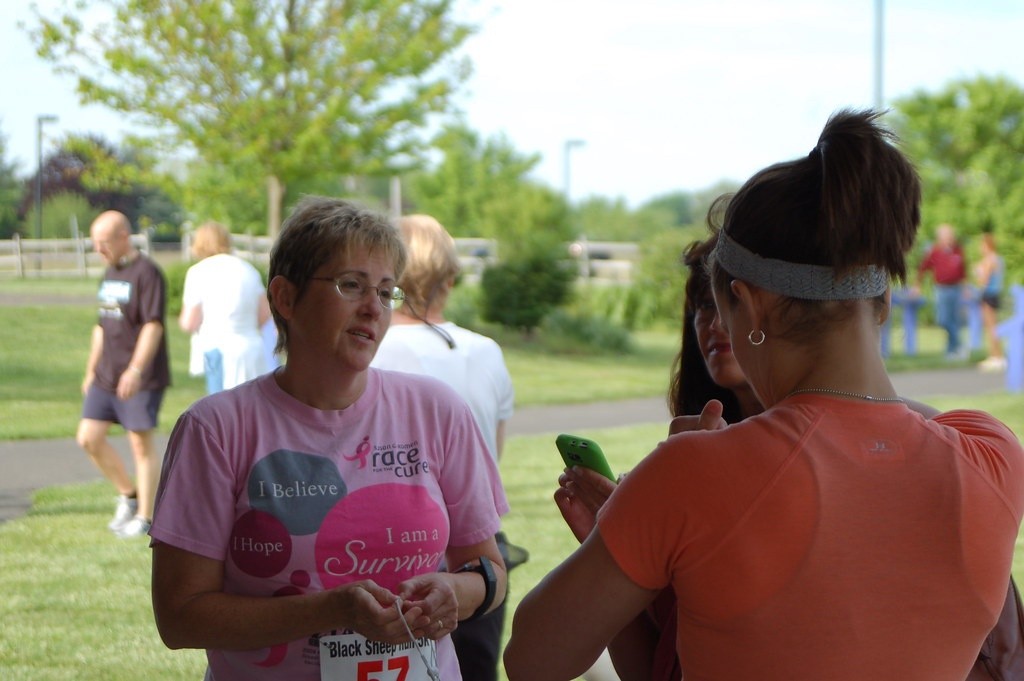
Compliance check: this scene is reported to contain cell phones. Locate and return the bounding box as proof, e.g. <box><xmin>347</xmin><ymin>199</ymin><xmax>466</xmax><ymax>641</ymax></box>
<box><xmin>555</xmin><ymin>433</ymin><xmax>617</xmax><ymax>484</ymax></box>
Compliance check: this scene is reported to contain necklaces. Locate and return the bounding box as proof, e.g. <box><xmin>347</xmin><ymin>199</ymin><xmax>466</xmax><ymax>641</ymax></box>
<box><xmin>795</xmin><ymin>389</ymin><xmax>902</xmax><ymax>402</ymax></box>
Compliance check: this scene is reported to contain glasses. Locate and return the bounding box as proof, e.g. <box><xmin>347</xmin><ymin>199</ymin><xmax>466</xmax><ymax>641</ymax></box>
<box><xmin>309</xmin><ymin>273</ymin><xmax>408</xmax><ymax>310</ymax></box>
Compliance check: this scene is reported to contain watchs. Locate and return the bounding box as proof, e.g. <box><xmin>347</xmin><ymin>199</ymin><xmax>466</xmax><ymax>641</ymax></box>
<box><xmin>451</xmin><ymin>556</ymin><xmax>497</xmax><ymax>623</ymax></box>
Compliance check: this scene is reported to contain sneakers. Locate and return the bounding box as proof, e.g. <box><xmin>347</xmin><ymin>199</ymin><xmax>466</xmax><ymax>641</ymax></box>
<box><xmin>108</xmin><ymin>495</ymin><xmax>137</xmax><ymax>529</ymax></box>
<box><xmin>117</xmin><ymin>516</ymin><xmax>152</xmax><ymax>539</ymax></box>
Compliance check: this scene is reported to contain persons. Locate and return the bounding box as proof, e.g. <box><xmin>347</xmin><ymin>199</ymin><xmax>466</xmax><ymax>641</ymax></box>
<box><xmin>503</xmin><ymin>108</ymin><xmax>1024</xmax><ymax>681</ymax></box>
<box><xmin>368</xmin><ymin>213</ymin><xmax>514</xmax><ymax>463</ymax></box>
<box><xmin>178</xmin><ymin>221</ymin><xmax>271</xmax><ymax>391</ymax></box>
<box><xmin>77</xmin><ymin>210</ymin><xmax>172</xmax><ymax>537</ymax></box>
<box><xmin>148</xmin><ymin>189</ymin><xmax>506</xmax><ymax>681</ymax></box>
<box><xmin>554</xmin><ymin>224</ymin><xmax>1024</xmax><ymax>681</ymax></box>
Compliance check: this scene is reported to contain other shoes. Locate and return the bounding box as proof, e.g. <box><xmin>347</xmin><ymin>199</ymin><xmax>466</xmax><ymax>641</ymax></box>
<box><xmin>980</xmin><ymin>357</ymin><xmax>1006</xmax><ymax>373</ymax></box>
